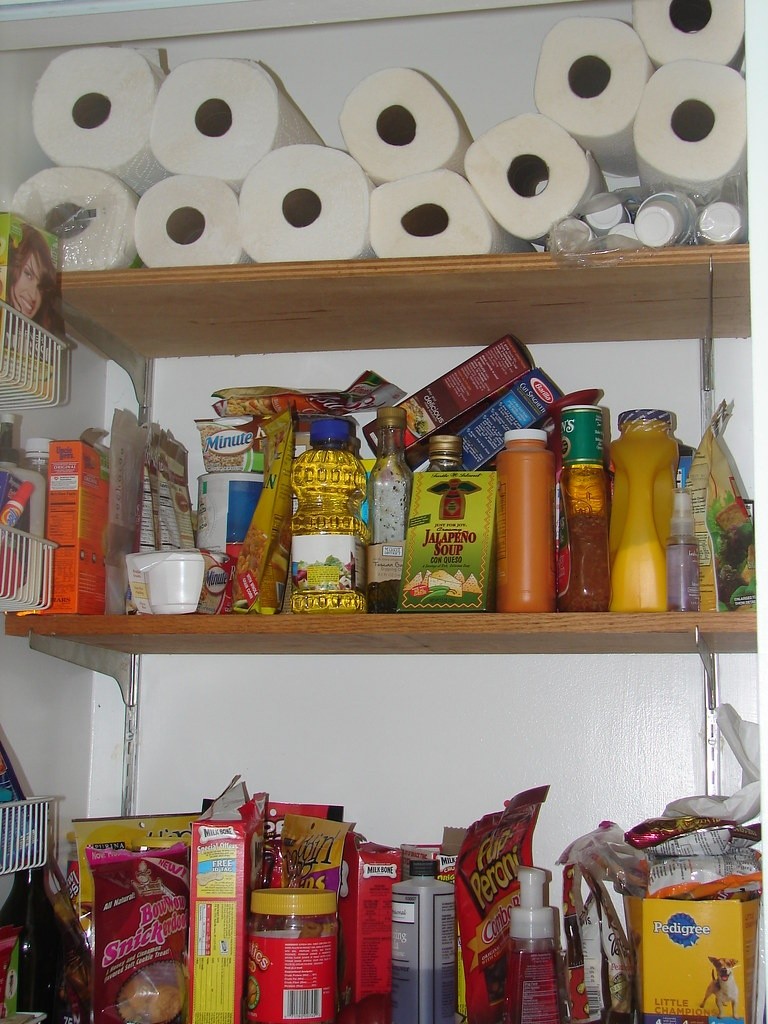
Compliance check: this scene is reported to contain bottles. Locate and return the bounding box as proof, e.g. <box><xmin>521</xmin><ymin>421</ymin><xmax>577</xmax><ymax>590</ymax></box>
<box><xmin>497</xmin><ymin>430</ymin><xmax>556</xmax><ymax>613</ymax></box>
<box><xmin>291</xmin><ymin>421</ymin><xmax>368</xmax><ymax>611</ymax></box>
<box><xmin>558</xmin><ymin>405</ymin><xmax>610</xmax><ymax>610</ymax></box>
<box><xmin>392</xmin><ymin>862</ymin><xmax>459</xmax><ymax>1024</ymax></box>
<box><xmin>0</xmin><ymin>414</ymin><xmax>19</xmax><ymax>469</ymax></box>
<box><xmin>429</xmin><ymin>435</ymin><xmax>466</xmax><ymax>473</ymax></box>
<box><xmin>611</xmin><ymin>409</ymin><xmax>679</xmax><ymax>612</ymax></box>
<box><xmin>667</xmin><ymin>489</ymin><xmax>700</xmax><ymax>612</ymax></box>
<box><xmin>369</xmin><ymin>408</ymin><xmax>412</xmax><ymax>611</ymax></box>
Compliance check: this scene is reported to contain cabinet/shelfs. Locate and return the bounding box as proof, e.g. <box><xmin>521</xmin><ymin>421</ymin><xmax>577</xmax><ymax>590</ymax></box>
<box><xmin>0</xmin><ymin>300</ymin><xmax>76</xmax><ymax>1024</ymax></box>
<box><xmin>2</xmin><ymin>241</ymin><xmax>754</xmax><ymax>710</ymax></box>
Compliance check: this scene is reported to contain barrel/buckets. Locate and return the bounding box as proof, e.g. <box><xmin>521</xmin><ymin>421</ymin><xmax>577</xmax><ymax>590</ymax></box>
<box><xmin>197</xmin><ymin>471</ymin><xmax>266</xmax><ymax>545</ymax></box>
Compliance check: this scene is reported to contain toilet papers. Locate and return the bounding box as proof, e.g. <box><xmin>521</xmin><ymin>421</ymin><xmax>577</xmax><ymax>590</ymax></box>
<box><xmin>466</xmin><ymin>115</ymin><xmax>607</xmax><ymax>241</ymax></box>
<box><xmin>238</xmin><ymin>139</ymin><xmax>369</xmax><ymax>263</ymax></box>
<box><xmin>371</xmin><ymin>178</ymin><xmax>524</xmax><ymax>263</ymax></box>
<box><xmin>538</xmin><ymin>20</ymin><xmax>648</xmax><ymax>178</ymax></box>
<box><xmin>136</xmin><ymin>173</ymin><xmax>238</xmax><ymax>266</ymax></box>
<box><xmin>12</xmin><ymin>166</ymin><xmax>138</xmax><ymax>271</ymax></box>
<box><xmin>629</xmin><ymin>0</ymin><xmax>748</xmax><ymax>71</ymax></box>
<box><xmin>635</xmin><ymin>61</ymin><xmax>750</xmax><ymax>200</ymax></box>
<box><xmin>346</xmin><ymin>62</ymin><xmax>471</xmax><ymax>186</ymax></box>
<box><xmin>153</xmin><ymin>59</ymin><xmax>330</xmax><ymax>183</ymax></box>
<box><xmin>31</xmin><ymin>45</ymin><xmax>165</xmax><ymax>188</ymax></box>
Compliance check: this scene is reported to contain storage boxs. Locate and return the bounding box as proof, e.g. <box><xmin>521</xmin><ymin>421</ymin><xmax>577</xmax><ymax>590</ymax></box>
<box><xmin>394</xmin><ymin>471</ymin><xmax>496</xmax><ymax>613</ymax></box>
<box><xmin>339</xmin><ymin>830</ymin><xmax>403</xmax><ymax>1005</ymax></box>
<box><xmin>0</xmin><ymin>924</ymin><xmax>23</xmax><ymax>1020</ymax></box>
<box><xmin>39</xmin><ymin>426</ymin><xmax>109</xmax><ymax>614</ymax></box>
<box><xmin>411</xmin><ymin>367</ymin><xmax>561</xmax><ymax>470</ymax></box>
<box><xmin>0</xmin><ymin>212</ymin><xmax>60</xmax><ymax>403</ymax></box>
<box><xmin>188</xmin><ymin>773</ymin><xmax>269</xmax><ymax>1024</ymax></box>
<box><xmin>400</xmin><ymin>826</ymin><xmax>468</xmax><ymax>884</ymax></box>
<box><xmin>361</xmin><ymin>334</ymin><xmax>535</xmax><ymax>470</ymax></box>
<box><xmin>622</xmin><ymin>894</ymin><xmax>759</xmax><ymax>1024</ymax></box>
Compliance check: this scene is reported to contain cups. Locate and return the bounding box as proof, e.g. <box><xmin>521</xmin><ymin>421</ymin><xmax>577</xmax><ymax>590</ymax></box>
<box><xmin>24</xmin><ymin>437</ymin><xmax>54</xmax><ymax>475</ymax></box>
